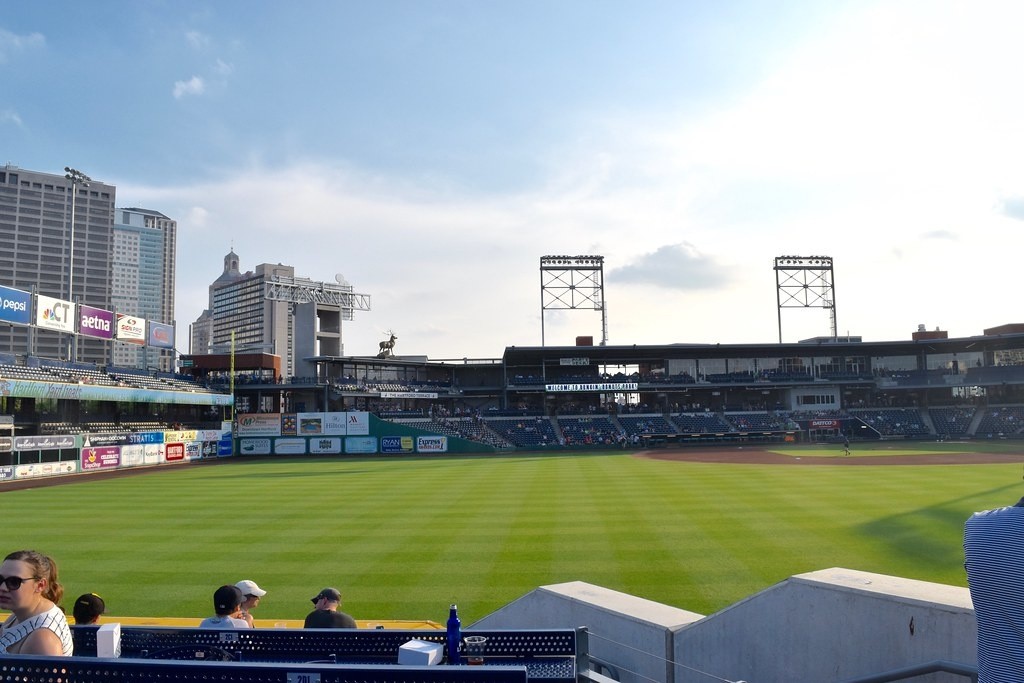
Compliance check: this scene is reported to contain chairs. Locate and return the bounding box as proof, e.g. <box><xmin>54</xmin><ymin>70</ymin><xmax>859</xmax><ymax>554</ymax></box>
<box><xmin>511</xmin><ymin>374</ymin><xmax>696</xmax><ymax>386</ymax></box>
<box><xmin>705</xmin><ymin>371</ymin><xmax>815</xmax><ymax>383</ymax></box>
<box><xmin>378</xmin><ymin>406</ymin><xmax>1024</xmax><ymax>448</ymax></box>
<box><xmin>0</xmin><ymin>363</ymin><xmax>224</xmax><ymax>393</ymax></box>
<box><xmin>329</xmin><ymin>377</ymin><xmax>448</xmax><ymax>393</ymax></box>
<box><xmin>40</xmin><ymin>422</ymin><xmax>206</xmax><ymax>435</ymax></box>
<box><xmin>820</xmin><ymin>369</ymin><xmax>911</xmax><ymax>385</ymax></box>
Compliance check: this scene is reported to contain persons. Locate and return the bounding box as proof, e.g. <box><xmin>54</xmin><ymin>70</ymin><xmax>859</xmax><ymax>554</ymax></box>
<box><xmin>235</xmin><ymin>580</ymin><xmax>266</xmax><ymax>628</ymax></box>
<box><xmin>349</xmin><ymin>373</ymin><xmax>352</xmax><ymax>379</ymax></box>
<box><xmin>843</xmin><ymin>436</ymin><xmax>850</xmax><ymax>455</ymax></box>
<box><xmin>335</xmin><ymin>383</ymin><xmax>365</xmax><ymax>391</ymax></box>
<box><xmin>751</xmin><ymin>368</ymin><xmax>768</xmax><ymax>381</ymax></box>
<box><xmin>199</xmin><ymin>584</ymin><xmax>250</xmax><ymax>628</ymax></box>
<box><xmin>174</xmin><ymin>423</ymin><xmax>181</xmax><ymax>431</ymax></box>
<box><xmin>206</xmin><ymin>371</ymin><xmax>258</xmax><ymax>384</ymax></box>
<box><xmin>343</xmin><ymin>401</ymin><xmax>424</xmax><ymax>418</ymax></box>
<box><xmin>69</xmin><ymin>373</ymin><xmax>95</xmax><ymax>386</ymax></box>
<box><xmin>304</xmin><ymin>588</ymin><xmax>357</xmax><ymax>628</ymax></box>
<box><xmin>93</xmin><ymin>360</ymin><xmax>97</xmax><ymax>365</ymax></box>
<box><xmin>153</xmin><ymin>373</ymin><xmax>157</xmax><ymax>380</ymax></box>
<box><xmin>0</xmin><ymin>550</ymin><xmax>73</xmax><ymax>683</ymax></box>
<box><xmin>508</xmin><ymin>402</ymin><xmax>700</xmax><ymax>447</ymax></box>
<box><xmin>435</xmin><ymin>416</ymin><xmax>506</xmax><ymax>448</ymax></box>
<box><xmin>72</xmin><ymin>592</ymin><xmax>106</xmax><ymax>626</ymax></box>
<box><xmin>962</xmin><ymin>497</ymin><xmax>1024</xmax><ymax>683</ymax></box>
<box><xmin>647</xmin><ymin>368</ymin><xmax>688</xmax><ymax>385</ymax></box>
<box><xmin>277</xmin><ymin>374</ymin><xmax>283</xmax><ymax>385</ymax></box>
<box><xmin>721</xmin><ymin>407</ymin><xmax>840</xmax><ymax>442</ymax></box>
<box><xmin>428</xmin><ymin>403</ymin><xmax>482</xmax><ymax>417</ymax></box>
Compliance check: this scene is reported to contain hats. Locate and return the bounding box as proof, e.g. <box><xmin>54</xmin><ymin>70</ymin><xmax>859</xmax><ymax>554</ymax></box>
<box><xmin>214</xmin><ymin>584</ymin><xmax>247</xmax><ymax>608</ymax></box>
<box><xmin>235</xmin><ymin>580</ymin><xmax>267</xmax><ymax>597</ymax></box>
<box><xmin>73</xmin><ymin>593</ymin><xmax>104</xmax><ymax>621</ymax></box>
<box><xmin>310</xmin><ymin>588</ymin><xmax>341</xmax><ymax>606</ymax></box>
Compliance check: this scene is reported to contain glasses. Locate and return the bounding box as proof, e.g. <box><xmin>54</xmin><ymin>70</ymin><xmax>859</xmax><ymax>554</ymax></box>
<box><xmin>0</xmin><ymin>574</ymin><xmax>38</xmax><ymax>590</ymax></box>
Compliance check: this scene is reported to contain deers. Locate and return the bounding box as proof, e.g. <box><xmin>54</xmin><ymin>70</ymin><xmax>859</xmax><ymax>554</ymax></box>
<box><xmin>379</xmin><ymin>329</ymin><xmax>397</xmax><ymax>355</ymax></box>
